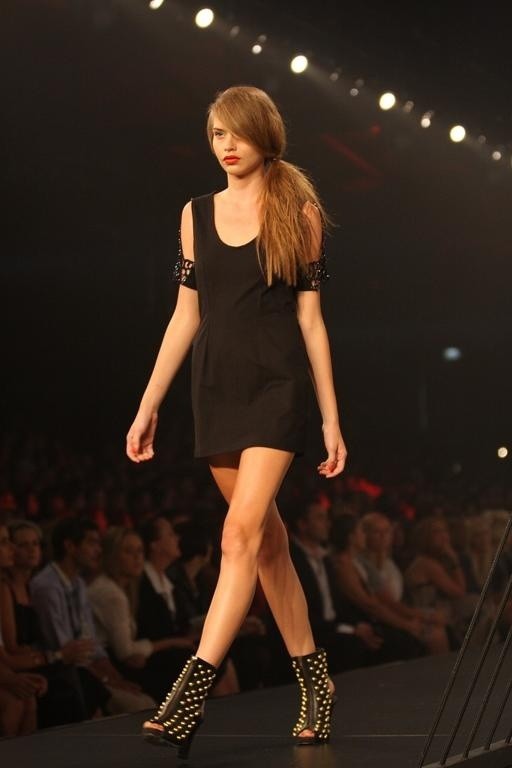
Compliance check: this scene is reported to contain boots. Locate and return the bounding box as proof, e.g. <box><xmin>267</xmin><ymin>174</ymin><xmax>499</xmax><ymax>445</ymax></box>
<box><xmin>143</xmin><ymin>655</ymin><xmax>217</xmax><ymax>757</ymax></box>
<box><xmin>288</xmin><ymin>648</ymin><xmax>336</xmax><ymax>745</ymax></box>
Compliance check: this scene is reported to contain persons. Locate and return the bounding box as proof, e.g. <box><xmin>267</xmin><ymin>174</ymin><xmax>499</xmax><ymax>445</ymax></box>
<box><xmin>125</xmin><ymin>85</ymin><xmax>348</xmax><ymax>759</ymax></box>
<box><xmin>0</xmin><ymin>466</ymin><xmax>512</xmax><ymax>736</ymax></box>
